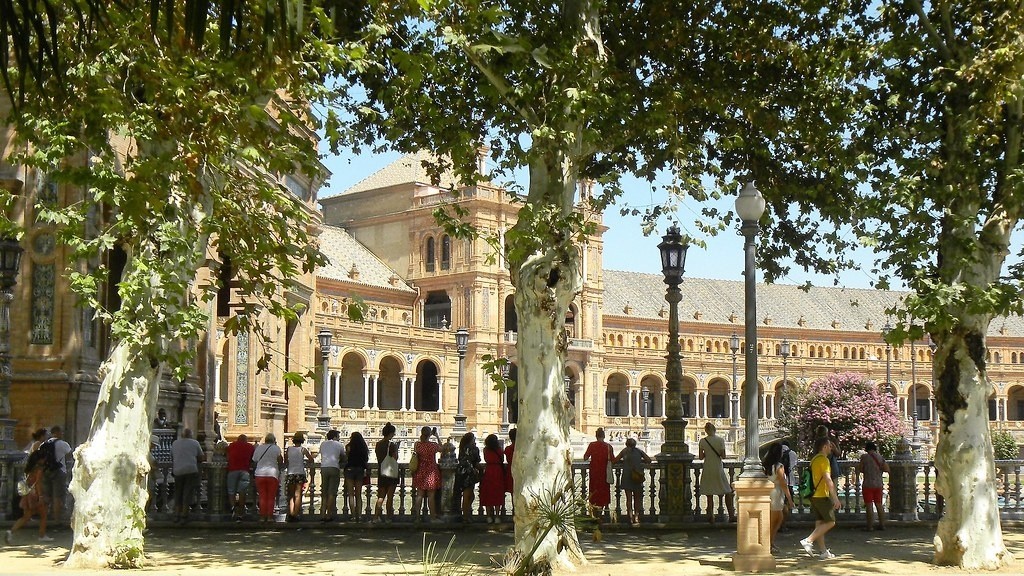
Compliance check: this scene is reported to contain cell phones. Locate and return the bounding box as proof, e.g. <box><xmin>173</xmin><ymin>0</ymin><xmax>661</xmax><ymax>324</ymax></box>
<box><xmin>433</xmin><ymin>427</ymin><xmax>436</xmax><ymax>435</ymax></box>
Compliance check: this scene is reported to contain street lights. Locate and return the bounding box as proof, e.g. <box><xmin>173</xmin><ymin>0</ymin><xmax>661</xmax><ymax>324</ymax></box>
<box><xmin>0</xmin><ymin>234</ymin><xmax>25</xmax><ymax>528</ymax></box>
<box><xmin>640</xmin><ymin>387</ymin><xmax>651</xmax><ymax>439</ymax></box>
<box><xmin>780</xmin><ymin>336</ymin><xmax>790</xmax><ymax>390</ymax></box>
<box><xmin>317</xmin><ymin>329</ymin><xmax>333</xmax><ymax>431</ymax></box>
<box><xmin>657</xmin><ymin>226</ymin><xmax>696</xmax><ymax>523</ymax></box>
<box><xmin>500</xmin><ymin>357</ymin><xmax>511</xmax><ymax>435</ymax></box>
<box><xmin>730</xmin><ymin>180</ymin><xmax>768</xmax><ymax>479</ymax></box>
<box><xmin>453</xmin><ymin>328</ymin><xmax>470</xmax><ymax>431</ymax></box>
<box><xmin>928</xmin><ymin>336</ymin><xmax>939</xmax><ymax>425</ymax></box>
<box><xmin>728</xmin><ymin>330</ymin><xmax>739</xmax><ymax>427</ymax></box>
<box><xmin>880</xmin><ymin>316</ymin><xmax>894</xmax><ymax>395</ymax></box>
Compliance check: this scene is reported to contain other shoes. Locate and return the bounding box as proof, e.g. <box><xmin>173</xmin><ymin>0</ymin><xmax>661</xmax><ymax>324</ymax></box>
<box><xmin>234</xmin><ymin>515</ymin><xmax>504</xmax><ymax>528</ymax></box>
<box><xmin>180</xmin><ymin>516</ymin><xmax>191</xmax><ymax>525</ymax></box>
<box><xmin>800</xmin><ymin>537</ymin><xmax>814</xmax><ymax>558</ymax></box>
<box><xmin>5</xmin><ymin>530</ymin><xmax>14</xmax><ymax>545</ymax></box>
<box><xmin>151</xmin><ymin>505</ymin><xmax>158</xmax><ymax>510</ymax></box>
<box><xmin>729</xmin><ymin>516</ymin><xmax>737</xmax><ymax>522</ymax></box>
<box><xmin>173</xmin><ymin>516</ymin><xmax>181</xmax><ymax>522</ymax></box>
<box><xmin>39</xmin><ymin>535</ymin><xmax>54</xmax><ymax>542</ymax></box>
<box><xmin>820</xmin><ymin>548</ymin><xmax>835</xmax><ymax>560</ymax></box>
<box><xmin>162</xmin><ymin>504</ymin><xmax>169</xmax><ymax>510</ymax></box>
<box><xmin>876</xmin><ymin>526</ymin><xmax>887</xmax><ymax>530</ymax></box>
<box><xmin>627</xmin><ymin>518</ymin><xmax>640</xmax><ymax>523</ymax></box>
<box><xmin>53</xmin><ymin>522</ymin><xmax>66</xmax><ymax>533</ymax></box>
<box><xmin>861</xmin><ymin>526</ymin><xmax>874</xmax><ymax>532</ymax></box>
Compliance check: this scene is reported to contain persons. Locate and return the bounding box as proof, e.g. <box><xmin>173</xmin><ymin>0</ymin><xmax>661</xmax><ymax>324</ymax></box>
<box><xmin>480</xmin><ymin>434</ymin><xmax>507</xmax><ymax>524</ymax></box>
<box><xmin>613</xmin><ymin>438</ymin><xmax>651</xmax><ymax>524</ymax></box>
<box><xmin>345</xmin><ymin>432</ymin><xmax>369</xmax><ymax>521</ymax></box>
<box><xmin>283</xmin><ymin>432</ymin><xmax>315</xmax><ymax>523</ymax></box>
<box><xmin>584</xmin><ymin>428</ymin><xmax>615</xmax><ymax>525</ymax></box>
<box><xmin>699</xmin><ymin>423</ymin><xmax>737</xmax><ymax>524</ymax></box>
<box><xmin>319</xmin><ymin>430</ymin><xmax>345</xmax><ymax>520</ymax></box>
<box><xmin>459</xmin><ymin>433</ymin><xmax>481</xmax><ymax>523</ymax></box>
<box><xmin>250</xmin><ymin>433</ymin><xmax>284</xmax><ymax>522</ymax></box>
<box><xmin>761</xmin><ymin>424</ymin><xmax>890</xmax><ymax>560</ymax></box>
<box><xmin>408</xmin><ymin>426</ymin><xmax>446</xmax><ymax>525</ymax></box>
<box><xmin>225</xmin><ymin>433</ymin><xmax>255</xmax><ymax>520</ymax></box>
<box><xmin>503</xmin><ymin>428</ymin><xmax>517</xmax><ymax>518</ymax></box>
<box><xmin>3</xmin><ymin>426</ymin><xmax>75</xmax><ymax>546</ymax></box>
<box><xmin>371</xmin><ymin>421</ymin><xmax>399</xmax><ymax>524</ymax></box>
<box><xmin>147</xmin><ymin>407</ymin><xmax>222</xmax><ymax>525</ymax></box>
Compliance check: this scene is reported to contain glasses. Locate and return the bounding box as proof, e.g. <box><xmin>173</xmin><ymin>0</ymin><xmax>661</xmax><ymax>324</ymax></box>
<box><xmin>153</xmin><ymin>444</ymin><xmax>157</xmax><ymax>447</ymax></box>
<box><xmin>150</xmin><ymin>435</ymin><xmax>160</xmax><ymax>446</ymax></box>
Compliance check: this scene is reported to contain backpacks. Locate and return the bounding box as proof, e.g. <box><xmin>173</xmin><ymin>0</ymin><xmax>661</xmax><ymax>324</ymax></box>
<box><xmin>39</xmin><ymin>439</ymin><xmax>64</xmax><ymax>472</ymax></box>
<box><xmin>17</xmin><ymin>479</ymin><xmax>31</xmax><ymax>496</ymax></box>
<box><xmin>780</xmin><ymin>451</ymin><xmax>791</xmax><ymax>476</ymax></box>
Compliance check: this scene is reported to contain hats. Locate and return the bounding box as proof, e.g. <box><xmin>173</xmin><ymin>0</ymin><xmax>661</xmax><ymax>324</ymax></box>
<box><xmin>292</xmin><ymin>432</ymin><xmax>305</xmax><ymax>441</ymax></box>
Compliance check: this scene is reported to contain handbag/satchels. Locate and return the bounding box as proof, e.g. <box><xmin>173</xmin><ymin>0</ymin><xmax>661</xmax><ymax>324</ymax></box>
<box><xmin>455</xmin><ymin>460</ymin><xmax>480</xmax><ymax>486</ymax></box>
<box><xmin>379</xmin><ymin>440</ymin><xmax>399</xmax><ymax>480</ymax></box>
<box><xmin>631</xmin><ymin>469</ymin><xmax>646</xmax><ymax>483</ymax></box>
<box><xmin>606</xmin><ymin>443</ymin><xmax>615</xmax><ymax>484</ymax></box>
<box><xmin>409</xmin><ymin>452</ymin><xmax>418</xmax><ymax>473</ymax></box>
